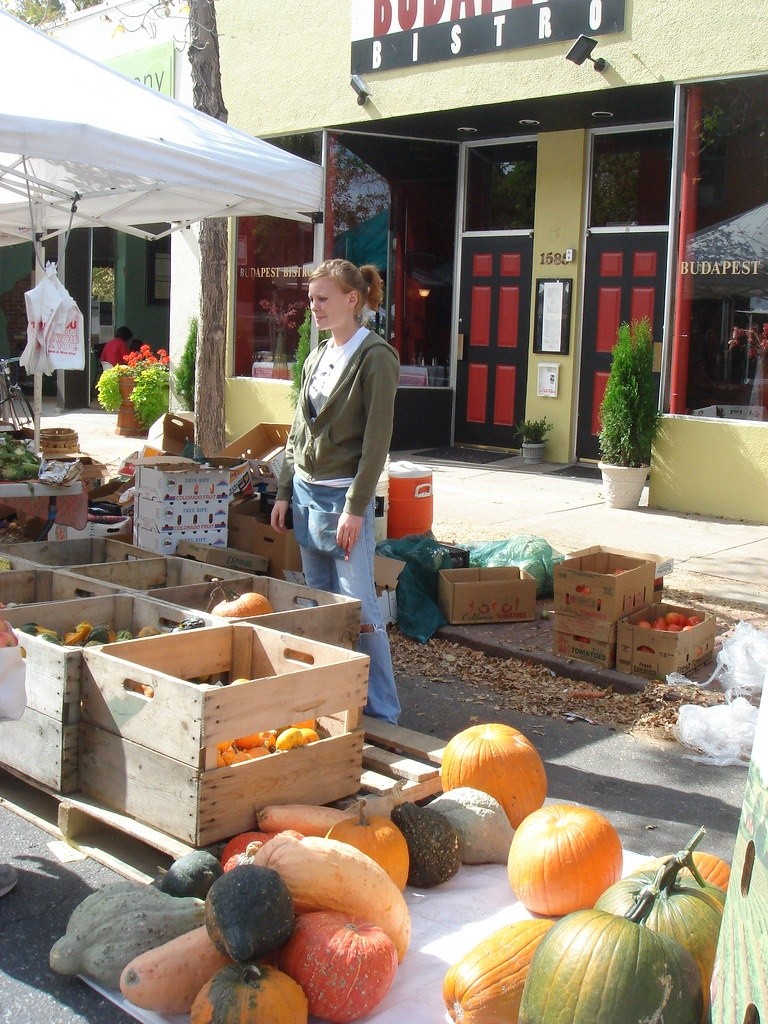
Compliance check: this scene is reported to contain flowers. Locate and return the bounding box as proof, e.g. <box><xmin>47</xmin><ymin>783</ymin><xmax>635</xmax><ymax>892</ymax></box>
<box><xmin>261</xmin><ymin>298</ymin><xmax>305</xmax><ymax>329</ymax></box>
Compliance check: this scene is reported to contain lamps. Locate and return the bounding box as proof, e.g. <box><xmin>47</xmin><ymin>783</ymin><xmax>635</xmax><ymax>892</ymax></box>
<box><xmin>418</xmin><ymin>287</ymin><xmax>431</xmax><ymax>297</ymax></box>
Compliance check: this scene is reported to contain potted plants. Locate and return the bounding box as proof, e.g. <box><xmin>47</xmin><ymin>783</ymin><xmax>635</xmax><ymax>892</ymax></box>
<box><xmin>94</xmin><ymin>362</ymin><xmax>169</xmax><ymax>438</ymax></box>
<box><xmin>598</xmin><ymin>316</ymin><xmax>659</xmax><ymax>510</ymax></box>
<box><xmin>517</xmin><ymin>419</ymin><xmax>553</xmax><ymax>464</ymax></box>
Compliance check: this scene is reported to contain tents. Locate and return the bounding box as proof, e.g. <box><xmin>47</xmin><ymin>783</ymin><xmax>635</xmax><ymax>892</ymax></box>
<box><xmin>0</xmin><ymin>7</ymin><xmax>323</xmax><ymax>453</ymax></box>
<box><xmin>684</xmin><ymin>203</ymin><xmax>768</xmax><ymax>382</ymax></box>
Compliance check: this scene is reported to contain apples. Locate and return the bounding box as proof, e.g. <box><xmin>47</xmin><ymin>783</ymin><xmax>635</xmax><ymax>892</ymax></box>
<box><xmin>565</xmin><ymin>568</ymin><xmax>700</xmax><ymax>654</ymax></box>
<box><xmin>0</xmin><ymin>617</ymin><xmax>18</xmax><ymax>648</ymax></box>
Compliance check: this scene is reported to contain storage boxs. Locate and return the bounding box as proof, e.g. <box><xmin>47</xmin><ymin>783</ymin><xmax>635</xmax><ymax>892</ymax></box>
<box><xmin>0</xmin><ymin>415</ymin><xmax>718</xmax><ymax>845</ymax></box>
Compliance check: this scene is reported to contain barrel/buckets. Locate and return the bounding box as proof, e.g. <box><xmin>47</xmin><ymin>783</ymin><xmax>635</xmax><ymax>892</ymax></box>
<box><xmin>372</xmin><ymin>454</ymin><xmax>434</xmax><ymax>548</ymax></box>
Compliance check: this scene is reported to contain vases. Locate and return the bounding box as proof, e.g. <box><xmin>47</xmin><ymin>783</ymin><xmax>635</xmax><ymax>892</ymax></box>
<box><xmin>272</xmin><ymin>334</ymin><xmax>289</xmax><ymax>378</ymax></box>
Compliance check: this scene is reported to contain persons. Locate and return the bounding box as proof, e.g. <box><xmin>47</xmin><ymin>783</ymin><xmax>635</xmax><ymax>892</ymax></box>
<box><xmin>101</xmin><ymin>326</ymin><xmax>132</xmax><ymax>367</ymax></box>
<box><xmin>270</xmin><ymin>258</ymin><xmax>402</xmax><ymax>758</ymax></box>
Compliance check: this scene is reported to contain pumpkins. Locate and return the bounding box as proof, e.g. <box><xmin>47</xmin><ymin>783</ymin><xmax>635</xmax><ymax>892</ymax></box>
<box><xmin>50</xmin><ymin>723</ymin><xmax>731</xmax><ymax>1024</ymax></box>
<box><xmin>18</xmin><ymin>578</ymin><xmax>320</xmax><ymax>768</ymax></box>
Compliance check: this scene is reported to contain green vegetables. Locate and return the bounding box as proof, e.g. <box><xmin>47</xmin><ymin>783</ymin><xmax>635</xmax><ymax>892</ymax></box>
<box><xmin>0</xmin><ymin>431</ymin><xmax>41</xmax><ymax>481</ymax></box>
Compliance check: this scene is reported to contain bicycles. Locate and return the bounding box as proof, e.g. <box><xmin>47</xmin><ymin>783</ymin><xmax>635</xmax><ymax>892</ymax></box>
<box><xmin>0</xmin><ymin>356</ymin><xmax>35</xmax><ymax>432</ymax></box>
